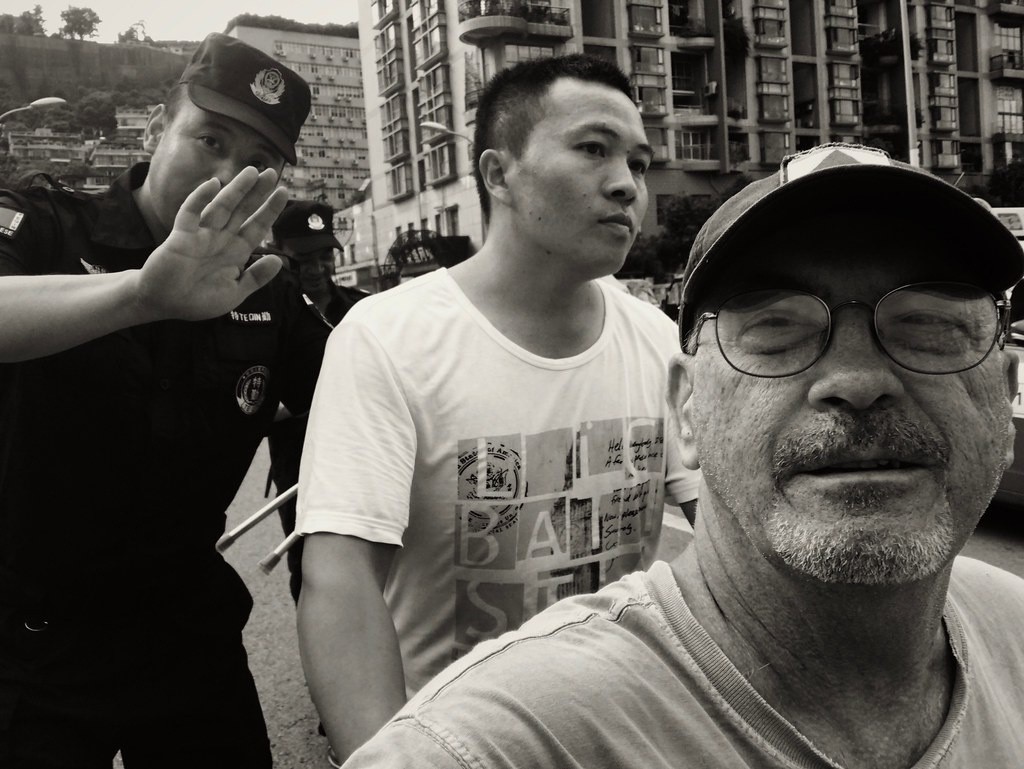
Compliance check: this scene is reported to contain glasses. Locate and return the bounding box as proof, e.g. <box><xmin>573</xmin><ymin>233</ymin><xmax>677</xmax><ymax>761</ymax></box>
<box><xmin>683</xmin><ymin>281</ymin><xmax>1011</xmax><ymax>378</ymax></box>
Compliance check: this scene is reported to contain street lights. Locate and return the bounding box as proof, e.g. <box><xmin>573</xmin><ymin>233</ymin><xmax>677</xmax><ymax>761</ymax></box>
<box><xmin>0</xmin><ymin>97</ymin><xmax>66</xmax><ymax>128</ymax></box>
<box><xmin>420</xmin><ymin>122</ymin><xmax>474</xmax><ymax>147</ymax></box>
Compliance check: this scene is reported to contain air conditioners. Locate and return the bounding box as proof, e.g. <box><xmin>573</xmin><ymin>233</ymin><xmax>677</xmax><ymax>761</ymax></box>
<box><xmin>275</xmin><ymin>49</ymin><xmax>368</xmax><ymax>201</ymax></box>
<box><xmin>704</xmin><ymin>81</ymin><xmax>718</xmax><ymax>97</ymax></box>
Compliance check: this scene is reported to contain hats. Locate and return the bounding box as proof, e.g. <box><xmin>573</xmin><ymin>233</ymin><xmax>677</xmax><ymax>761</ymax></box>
<box><xmin>272</xmin><ymin>200</ymin><xmax>344</xmax><ymax>252</ymax></box>
<box><xmin>680</xmin><ymin>141</ymin><xmax>1024</xmax><ymax>352</ymax></box>
<box><xmin>180</xmin><ymin>33</ymin><xmax>311</xmax><ymax>167</ymax></box>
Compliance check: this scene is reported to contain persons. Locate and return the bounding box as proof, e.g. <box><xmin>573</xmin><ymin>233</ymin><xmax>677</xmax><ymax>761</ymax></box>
<box><xmin>0</xmin><ymin>31</ymin><xmax>310</xmax><ymax>769</ymax></box>
<box><xmin>342</xmin><ymin>141</ymin><xmax>1023</xmax><ymax>768</ymax></box>
<box><xmin>298</xmin><ymin>56</ymin><xmax>698</xmax><ymax>766</ymax></box>
<box><xmin>264</xmin><ymin>199</ymin><xmax>373</xmax><ymax>607</ymax></box>
